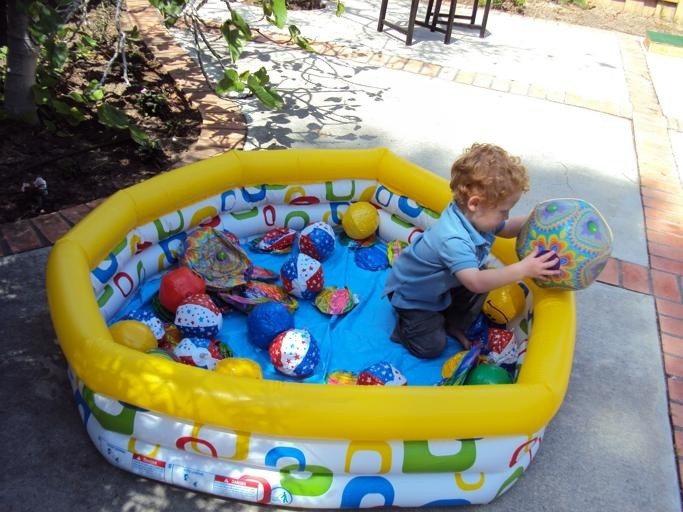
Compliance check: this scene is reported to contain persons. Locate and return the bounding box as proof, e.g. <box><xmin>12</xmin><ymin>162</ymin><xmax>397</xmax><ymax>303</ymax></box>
<box><xmin>381</xmin><ymin>142</ymin><xmax>560</xmax><ymax>360</ymax></box>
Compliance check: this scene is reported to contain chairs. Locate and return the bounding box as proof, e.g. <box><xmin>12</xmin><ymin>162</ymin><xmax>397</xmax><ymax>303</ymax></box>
<box><xmin>377</xmin><ymin>0</ymin><xmax>490</xmax><ymax>47</ymax></box>
